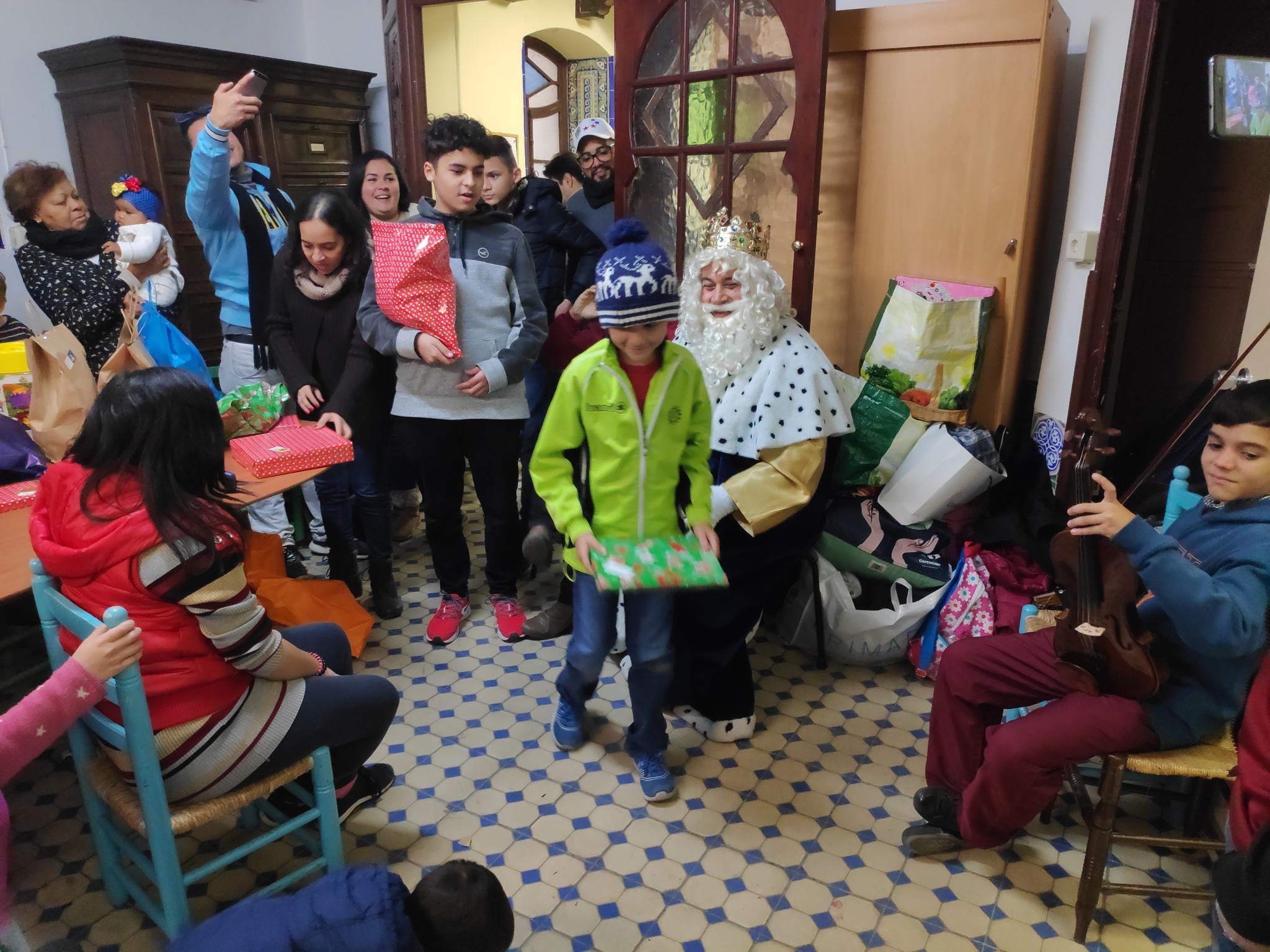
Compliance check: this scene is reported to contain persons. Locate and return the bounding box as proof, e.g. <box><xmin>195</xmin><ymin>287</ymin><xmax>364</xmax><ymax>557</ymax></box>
<box><xmin>897</xmin><ymin>382</ymin><xmax>1269</xmax><ymax>855</ymax></box>
<box><xmin>0</xmin><ymin>82</ymin><xmax>859</xmax><ymax>952</ymax></box>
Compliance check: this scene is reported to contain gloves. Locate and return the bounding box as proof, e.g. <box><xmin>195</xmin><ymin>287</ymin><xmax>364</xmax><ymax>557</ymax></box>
<box><xmin>710</xmin><ymin>484</ymin><xmax>733</xmax><ymax>530</ymax></box>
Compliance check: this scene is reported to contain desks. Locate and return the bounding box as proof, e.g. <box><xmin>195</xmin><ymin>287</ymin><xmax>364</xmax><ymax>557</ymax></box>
<box><xmin>0</xmin><ymin>420</ymin><xmax>329</xmax><ymax>693</ymax></box>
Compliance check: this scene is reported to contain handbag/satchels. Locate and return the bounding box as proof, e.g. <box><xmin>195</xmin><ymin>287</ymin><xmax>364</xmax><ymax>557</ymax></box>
<box><xmin>777</xmin><ymin>545</ymin><xmax>956</xmax><ymax>670</ymax></box>
<box><xmin>820</xmin><ymin>278</ymin><xmax>994</xmax><ymax>485</ymax></box>
<box><xmin>876</xmin><ymin>420</ymin><xmax>1008</xmax><ymax>526</ymax></box>
<box><xmin>96</xmin><ymin>277</ymin><xmax>224</xmax><ymax>398</ymax></box>
<box><xmin>23</xmin><ymin>299</ymin><xmax>96</xmax><ymax>463</ymax></box>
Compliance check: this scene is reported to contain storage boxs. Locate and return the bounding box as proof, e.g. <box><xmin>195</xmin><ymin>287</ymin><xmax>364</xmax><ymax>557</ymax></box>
<box><xmin>0</xmin><ymin>481</ymin><xmax>39</xmax><ymax>514</ymax></box>
<box><xmin>230</xmin><ymin>414</ymin><xmax>354</xmax><ymax>479</ymax></box>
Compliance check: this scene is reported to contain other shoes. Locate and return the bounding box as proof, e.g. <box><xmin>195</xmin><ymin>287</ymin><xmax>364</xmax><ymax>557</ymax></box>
<box><xmin>259</xmin><ymin>763</ymin><xmax>395</xmax><ymax>827</ymax></box>
<box><xmin>522</xmin><ymin>523</ymin><xmax>551</xmax><ymax>574</ymax></box>
<box><xmin>902</xmin><ymin>786</ymin><xmax>962</xmax><ymax>853</ymax></box>
<box><xmin>521</xmin><ymin>600</ymin><xmax>573</xmax><ymax>640</ymax></box>
<box><xmin>390</xmin><ymin>486</ymin><xmax>422</xmax><ymax>540</ymax></box>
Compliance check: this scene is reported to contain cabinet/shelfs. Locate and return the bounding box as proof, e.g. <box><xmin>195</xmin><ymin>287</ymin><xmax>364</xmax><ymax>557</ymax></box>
<box><xmin>35</xmin><ymin>33</ymin><xmax>378</xmax><ymax>365</ymax></box>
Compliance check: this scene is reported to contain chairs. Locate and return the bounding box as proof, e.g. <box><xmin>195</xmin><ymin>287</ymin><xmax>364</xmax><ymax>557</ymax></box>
<box><xmin>30</xmin><ymin>557</ymin><xmax>344</xmax><ymax>943</ymax></box>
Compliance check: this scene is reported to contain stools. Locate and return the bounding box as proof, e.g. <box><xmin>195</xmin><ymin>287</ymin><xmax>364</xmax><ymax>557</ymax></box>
<box><xmin>1040</xmin><ymin>719</ymin><xmax>1239</xmax><ymax>944</ymax></box>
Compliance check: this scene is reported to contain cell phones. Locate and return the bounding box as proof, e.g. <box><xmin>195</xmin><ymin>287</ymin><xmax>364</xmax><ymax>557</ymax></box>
<box><xmin>240</xmin><ymin>68</ymin><xmax>269</xmax><ymax>99</ymax></box>
<box><xmin>1207</xmin><ymin>53</ymin><xmax>1270</xmax><ymax>139</ymax></box>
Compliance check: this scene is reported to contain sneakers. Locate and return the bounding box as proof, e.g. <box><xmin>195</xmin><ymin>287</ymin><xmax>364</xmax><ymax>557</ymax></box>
<box><xmin>552</xmin><ymin>696</ymin><xmax>584</xmax><ymax>749</ymax></box>
<box><xmin>624</xmin><ymin>737</ymin><xmax>677</xmax><ymax>802</ymax></box>
<box><xmin>309</xmin><ymin>537</ymin><xmax>368</xmax><ymax>559</ymax></box>
<box><xmin>362</xmin><ymin>566</ymin><xmax>403</xmax><ymax>619</ymax></box>
<box><xmin>484</xmin><ymin>594</ymin><xmax>525</xmax><ymax>641</ymax></box>
<box><xmin>282</xmin><ymin>545</ymin><xmax>309</xmax><ymax>580</ymax></box>
<box><xmin>323</xmin><ymin>554</ymin><xmax>365</xmax><ymax>599</ymax></box>
<box><xmin>425</xmin><ymin>590</ymin><xmax>471</xmax><ymax>644</ymax></box>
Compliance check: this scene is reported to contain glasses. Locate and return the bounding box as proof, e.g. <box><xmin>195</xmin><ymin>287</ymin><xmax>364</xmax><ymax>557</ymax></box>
<box><xmin>577</xmin><ymin>142</ymin><xmax>615</xmax><ymax>170</ymax></box>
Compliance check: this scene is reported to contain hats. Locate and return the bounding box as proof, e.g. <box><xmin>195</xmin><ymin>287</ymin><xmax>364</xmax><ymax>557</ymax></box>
<box><xmin>572</xmin><ymin>117</ymin><xmax>614</xmax><ymax>149</ymax></box>
<box><xmin>594</xmin><ymin>217</ymin><xmax>680</xmax><ymax>329</ymax></box>
